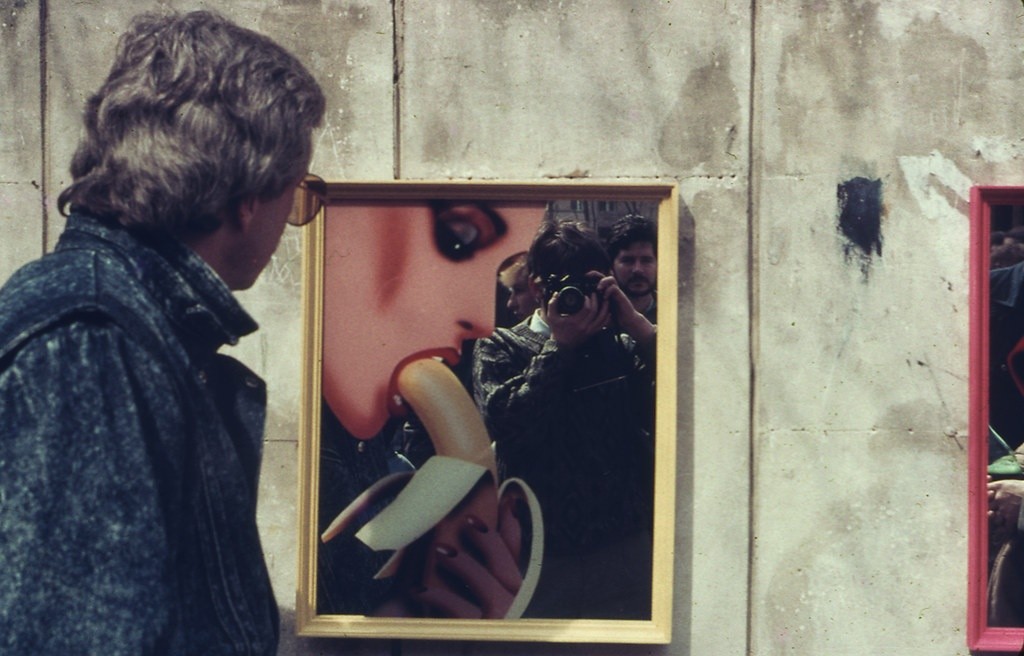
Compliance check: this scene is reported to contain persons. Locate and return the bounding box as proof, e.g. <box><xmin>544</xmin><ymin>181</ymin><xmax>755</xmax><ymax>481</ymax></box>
<box><xmin>469</xmin><ymin>211</ymin><xmax>658</xmax><ymax>624</ymax></box>
<box><xmin>0</xmin><ymin>9</ymin><xmax>328</xmax><ymax>656</ymax></box>
<box><xmin>986</xmin><ymin>440</ymin><xmax>1024</xmax><ymax>534</ymax></box>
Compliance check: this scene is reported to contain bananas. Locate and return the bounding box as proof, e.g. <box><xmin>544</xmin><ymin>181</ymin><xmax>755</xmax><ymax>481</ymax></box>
<box><xmin>319</xmin><ymin>361</ymin><xmax>543</xmax><ymax>621</ymax></box>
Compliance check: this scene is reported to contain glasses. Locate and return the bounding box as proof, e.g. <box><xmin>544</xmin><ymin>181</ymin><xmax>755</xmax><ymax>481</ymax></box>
<box><xmin>285</xmin><ymin>171</ymin><xmax>327</xmax><ymax>227</ymax></box>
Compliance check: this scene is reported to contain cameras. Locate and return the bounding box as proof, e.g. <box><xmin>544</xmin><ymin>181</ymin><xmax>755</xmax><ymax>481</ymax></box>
<box><xmin>544</xmin><ymin>273</ymin><xmax>619</xmax><ymax>330</ymax></box>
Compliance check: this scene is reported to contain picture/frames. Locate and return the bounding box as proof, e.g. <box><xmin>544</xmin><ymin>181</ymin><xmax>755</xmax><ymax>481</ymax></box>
<box><xmin>294</xmin><ymin>179</ymin><xmax>682</xmax><ymax>645</ymax></box>
<box><xmin>967</xmin><ymin>183</ymin><xmax>1024</xmax><ymax>655</ymax></box>
